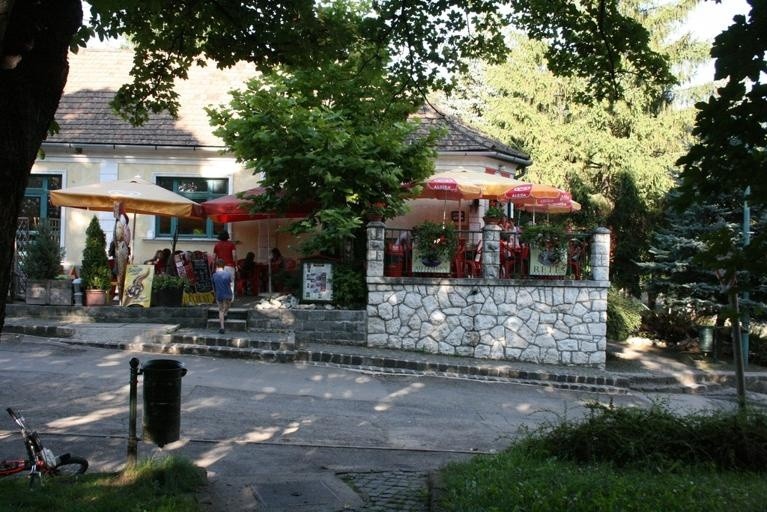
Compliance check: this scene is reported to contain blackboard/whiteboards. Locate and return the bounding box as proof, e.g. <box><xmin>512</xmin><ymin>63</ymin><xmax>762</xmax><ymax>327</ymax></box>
<box><xmin>190</xmin><ymin>250</ymin><xmax>213</xmax><ymax>295</ymax></box>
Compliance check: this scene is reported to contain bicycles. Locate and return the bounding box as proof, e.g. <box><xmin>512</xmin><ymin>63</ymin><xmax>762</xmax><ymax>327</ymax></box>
<box><xmin>0</xmin><ymin>406</ymin><xmax>89</xmax><ymax>492</ymax></box>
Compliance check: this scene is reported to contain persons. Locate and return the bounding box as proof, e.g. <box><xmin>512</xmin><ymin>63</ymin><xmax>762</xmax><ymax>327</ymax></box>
<box><xmin>240</xmin><ymin>252</ymin><xmax>263</xmax><ymax>297</ymax></box>
<box><xmin>210</xmin><ymin>258</ymin><xmax>233</xmax><ymax>333</ymax></box>
<box><xmin>475</xmin><ymin>213</ymin><xmax>521</xmax><ymax>279</ymax></box>
<box><xmin>395</xmin><ymin>226</ymin><xmax>418</xmax><ymax>264</ymax></box>
<box><xmin>210</xmin><ymin>229</ymin><xmax>239</xmax><ymax>305</ymax></box>
<box><xmin>261</xmin><ymin>247</ymin><xmax>287</xmax><ymax>291</ymax></box>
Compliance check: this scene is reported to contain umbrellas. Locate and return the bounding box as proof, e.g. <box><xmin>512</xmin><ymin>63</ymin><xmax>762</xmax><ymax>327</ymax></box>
<box><xmin>400</xmin><ymin>166</ymin><xmax>531</xmax><ymax>238</ymax></box>
<box><xmin>498</xmin><ymin>183</ymin><xmax>571</xmax><ymax>224</ymax></box>
<box><xmin>200</xmin><ymin>182</ymin><xmax>322</xmax><ymax>291</ymax></box>
<box><xmin>49</xmin><ymin>175</ymin><xmax>204</xmax><ymax>265</ymax></box>
<box><xmin>518</xmin><ymin>200</ymin><xmax>581</xmax><ymax>222</ymax></box>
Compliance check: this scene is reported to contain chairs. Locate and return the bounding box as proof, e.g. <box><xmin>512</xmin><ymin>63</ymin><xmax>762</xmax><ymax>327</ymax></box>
<box><xmin>453</xmin><ymin>238</ymin><xmax>587</xmax><ymax>281</ymax></box>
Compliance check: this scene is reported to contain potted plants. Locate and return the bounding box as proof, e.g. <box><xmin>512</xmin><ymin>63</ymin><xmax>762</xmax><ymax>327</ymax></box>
<box><xmin>151</xmin><ymin>272</ymin><xmax>193</xmax><ymax>307</ymax></box>
<box><xmin>80</xmin><ymin>213</ymin><xmax>113</xmax><ymax>306</ymax></box>
<box><xmin>482</xmin><ymin>206</ymin><xmax>502</xmax><ymax>224</ymax></box>
<box><xmin>21</xmin><ymin>217</ymin><xmax>74</xmax><ymax>306</ymax></box>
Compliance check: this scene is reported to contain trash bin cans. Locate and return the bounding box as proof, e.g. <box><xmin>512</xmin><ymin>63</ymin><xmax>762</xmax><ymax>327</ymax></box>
<box><xmin>140</xmin><ymin>358</ymin><xmax>187</xmax><ymax>448</ymax></box>
<box><xmin>698</xmin><ymin>326</ymin><xmax>713</xmax><ymax>354</ymax></box>
